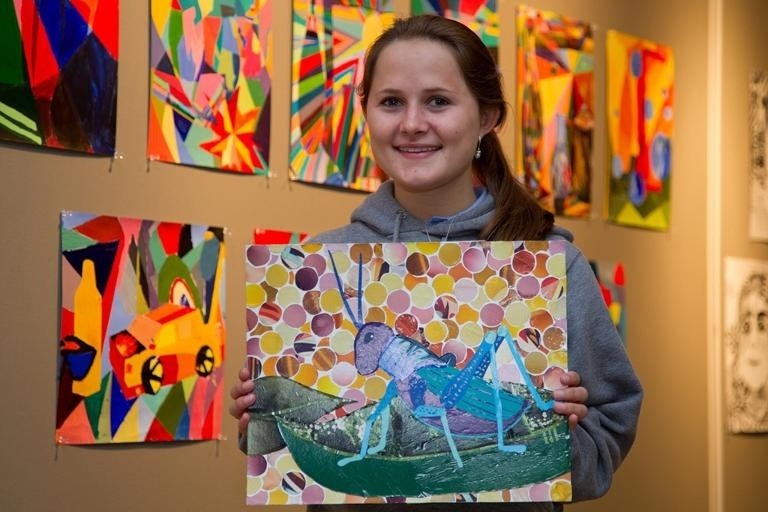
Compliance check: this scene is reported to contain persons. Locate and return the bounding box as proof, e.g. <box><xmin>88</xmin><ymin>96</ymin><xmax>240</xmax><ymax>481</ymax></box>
<box><xmin>728</xmin><ymin>270</ymin><xmax>766</xmax><ymax>432</ymax></box>
<box><xmin>227</xmin><ymin>15</ymin><xmax>646</xmax><ymax>511</ymax></box>
